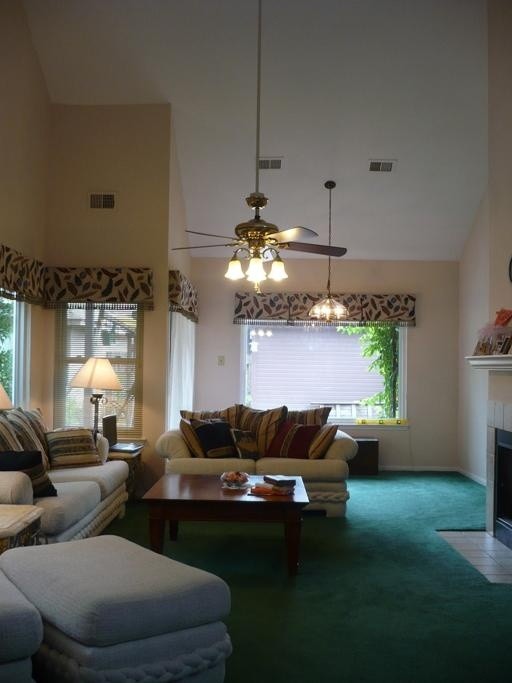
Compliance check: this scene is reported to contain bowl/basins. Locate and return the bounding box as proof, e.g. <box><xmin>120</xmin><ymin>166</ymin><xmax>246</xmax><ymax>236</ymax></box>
<box><xmin>220</xmin><ymin>472</ymin><xmax>248</xmax><ymax>486</ymax></box>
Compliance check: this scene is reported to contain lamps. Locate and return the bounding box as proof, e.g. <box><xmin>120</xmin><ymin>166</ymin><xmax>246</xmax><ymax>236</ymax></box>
<box><xmin>308</xmin><ymin>180</ymin><xmax>350</xmax><ymax>322</ymax></box>
<box><xmin>224</xmin><ymin>243</ymin><xmax>289</xmax><ymax>284</ymax></box>
<box><xmin>69</xmin><ymin>357</ymin><xmax>123</xmax><ymax>442</ymax></box>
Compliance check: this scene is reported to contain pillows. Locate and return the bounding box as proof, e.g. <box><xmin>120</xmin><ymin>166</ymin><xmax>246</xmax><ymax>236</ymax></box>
<box><xmin>179</xmin><ymin>403</ymin><xmax>339</xmax><ymax>459</ymax></box>
<box><xmin>0</xmin><ymin>406</ymin><xmax>104</xmax><ymax>499</ymax></box>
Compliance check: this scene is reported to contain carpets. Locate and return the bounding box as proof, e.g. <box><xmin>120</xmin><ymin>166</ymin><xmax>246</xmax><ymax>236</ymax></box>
<box><xmin>95</xmin><ymin>470</ymin><xmax>511</xmax><ymax>682</ymax></box>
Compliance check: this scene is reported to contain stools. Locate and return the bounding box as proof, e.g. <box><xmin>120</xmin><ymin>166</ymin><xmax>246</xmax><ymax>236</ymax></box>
<box><xmin>0</xmin><ymin>534</ymin><xmax>234</xmax><ymax>683</ymax></box>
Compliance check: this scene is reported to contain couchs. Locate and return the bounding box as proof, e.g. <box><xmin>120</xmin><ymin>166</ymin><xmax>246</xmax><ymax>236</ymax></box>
<box><xmin>0</xmin><ymin>426</ymin><xmax>131</xmax><ymax>545</ymax></box>
<box><xmin>155</xmin><ymin>429</ymin><xmax>359</xmax><ymax>518</ymax></box>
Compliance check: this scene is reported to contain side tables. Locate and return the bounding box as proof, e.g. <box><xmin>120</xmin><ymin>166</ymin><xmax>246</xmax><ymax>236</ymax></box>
<box><xmin>106</xmin><ymin>447</ymin><xmax>146</xmax><ymax>506</ymax></box>
<box><xmin>0</xmin><ymin>503</ymin><xmax>44</xmax><ymax>555</ymax></box>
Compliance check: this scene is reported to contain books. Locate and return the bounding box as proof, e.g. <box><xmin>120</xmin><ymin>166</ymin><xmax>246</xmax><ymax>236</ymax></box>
<box><xmin>251</xmin><ymin>475</ymin><xmax>296</xmax><ymax>496</ymax></box>
<box><xmin>472</xmin><ymin>332</ymin><xmax>512</xmax><ymax>355</ymax></box>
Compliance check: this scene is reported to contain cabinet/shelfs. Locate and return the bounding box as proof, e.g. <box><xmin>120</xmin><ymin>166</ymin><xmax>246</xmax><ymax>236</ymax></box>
<box><xmin>347</xmin><ymin>437</ymin><xmax>379</xmax><ymax>475</ymax></box>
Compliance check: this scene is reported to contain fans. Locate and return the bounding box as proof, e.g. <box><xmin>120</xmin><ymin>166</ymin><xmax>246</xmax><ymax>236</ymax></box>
<box><xmin>170</xmin><ymin>0</ymin><xmax>347</xmax><ymax>258</ymax></box>
